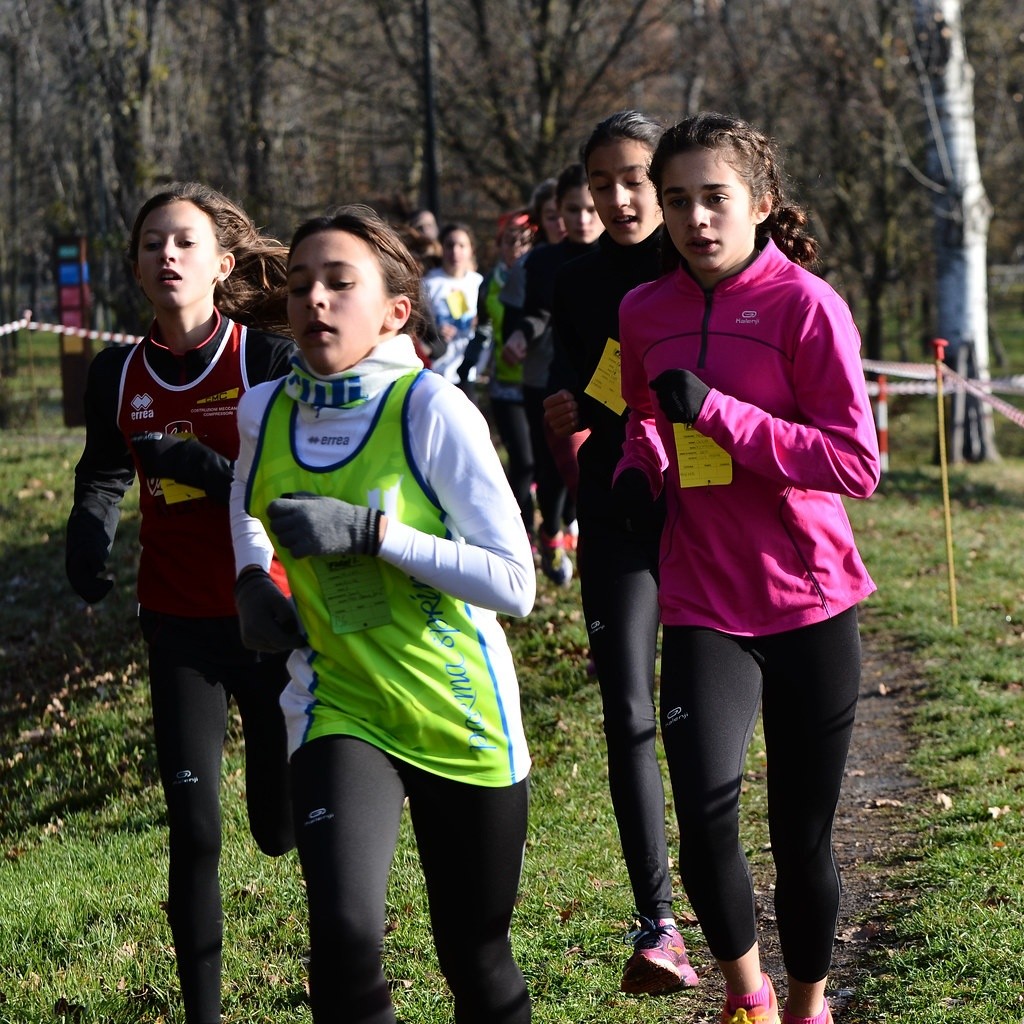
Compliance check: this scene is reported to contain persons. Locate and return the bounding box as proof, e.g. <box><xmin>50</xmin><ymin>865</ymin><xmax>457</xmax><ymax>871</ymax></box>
<box><xmin>523</xmin><ymin>110</ymin><xmax>709</xmax><ymax>1002</ymax></box>
<box><xmin>220</xmin><ymin>209</ymin><xmax>541</xmax><ymax>1024</ymax></box>
<box><xmin>63</xmin><ymin>174</ymin><xmax>307</xmax><ymax>1023</ymax></box>
<box><xmin>399</xmin><ymin>149</ymin><xmax>610</xmax><ymax>590</ymax></box>
<box><xmin>590</xmin><ymin>108</ymin><xmax>887</xmax><ymax>1024</ymax></box>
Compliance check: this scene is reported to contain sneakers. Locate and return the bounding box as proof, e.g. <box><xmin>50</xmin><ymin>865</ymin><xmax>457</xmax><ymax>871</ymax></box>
<box><xmin>719</xmin><ymin>973</ymin><xmax>835</xmax><ymax>1024</ymax></box>
<box><xmin>537</xmin><ymin>523</ymin><xmax>574</xmax><ymax>586</ymax></box>
<box><xmin>620</xmin><ymin>917</ymin><xmax>701</xmax><ymax>995</ymax></box>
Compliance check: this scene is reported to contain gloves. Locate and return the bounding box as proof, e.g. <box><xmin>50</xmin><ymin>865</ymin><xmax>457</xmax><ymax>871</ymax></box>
<box><xmin>128</xmin><ymin>432</ymin><xmax>187</xmax><ymax>481</ymax></box>
<box><xmin>611</xmin><ymin>467</ymin><xmax>653</xmax><ymax>532</ymax></box>
<box><xmin>65</xmin><ymin>542</ymin><xmax>114</xmax><ymax>605</ymax></box>
<box><xmin>648</xmin><ymin>366</ymin><xmax>710</xmax><ymax>426</ymax></box>
<box><xmin>232</xmin><ymin>569</ymin><xmax>307</xmax><ymax>662</ymax></box>
<box><xmin>264</xmin><ymin>488</ymin><xmax>386</xmax><ymax>559</ymax></box>
